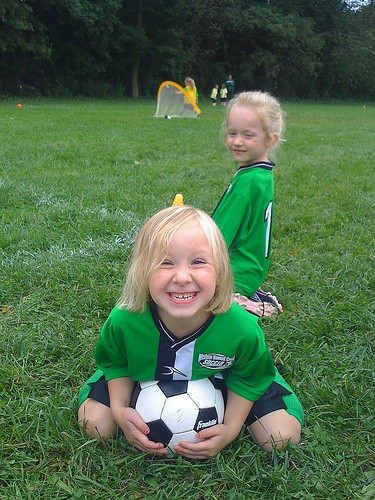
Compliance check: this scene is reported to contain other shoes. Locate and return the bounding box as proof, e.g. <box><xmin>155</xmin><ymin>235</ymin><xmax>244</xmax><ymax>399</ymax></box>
<box><xmin>248</xmin><ymin>288</ymin><xmax>283</xmax><ymax>314</ymax></box>
<box><xmin>233</xmin><ymin>293</ymin><xmax>278</xmax><ymax>318</ymax></box>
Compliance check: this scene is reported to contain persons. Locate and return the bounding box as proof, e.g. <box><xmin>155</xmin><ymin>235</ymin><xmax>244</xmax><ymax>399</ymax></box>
<box><xmin>224</xmin><ymin>73</ymin><xmax>236</xmax><ymax>99</ymax></box>
<box><xmin>76</xmin><ymin>205</ymin><xmax>304</xmax><ymax>458</ymax></box>
<box><xmin>209</xmin><ymin>90</ymin><xmax>284</xmax><ymax>320</ymax></box>
<box><xmin>220</xmin><ymin>84</ymin><xmax>228</xmax><ymax>107</ymax></box>
<box><xmin>173</xmin><ymin>77</ymin><xmax>199</xmax><ymax>118</ymax></box>
<box><xmin>210</xmin><ymin>84</ymin><xmax>218</xmax><ymax>106</ymax></box>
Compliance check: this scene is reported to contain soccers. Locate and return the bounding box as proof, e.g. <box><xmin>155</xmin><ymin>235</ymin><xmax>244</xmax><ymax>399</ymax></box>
<box><xmin>128</xmin><ymin>377</ymin><xmax>226</xmax><ymax>456</ymax></box>
